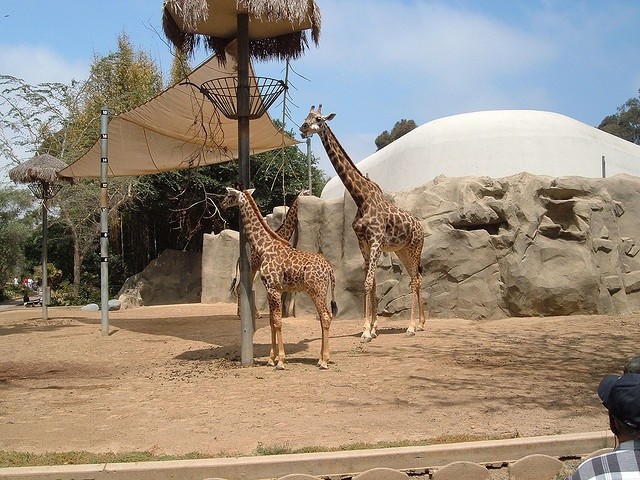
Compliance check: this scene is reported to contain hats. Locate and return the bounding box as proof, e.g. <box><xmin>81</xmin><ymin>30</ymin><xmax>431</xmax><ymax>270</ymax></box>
<box><xmin>598</xmin><ymin>373</ymin><xmax>640</xmax><ymax>427</ymax></box>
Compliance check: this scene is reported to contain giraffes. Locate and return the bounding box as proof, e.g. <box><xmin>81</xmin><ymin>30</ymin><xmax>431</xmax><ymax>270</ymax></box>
<box><xmin>221</xmin><ymin>188</ymin><xmax>341</xmax><ymax>371</ymax></box>
<box><xmin>298</xmin><ymin>101</ymin><xmax>430</xmax><ymax>344</ymax></box>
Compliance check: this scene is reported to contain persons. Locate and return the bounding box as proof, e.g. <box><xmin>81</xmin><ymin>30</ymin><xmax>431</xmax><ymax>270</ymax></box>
<box><xmin>14</xmin><ymin>272</ymin><xmax>33</xmax><ymax>287</ymax></box>
<box><xmin>570</xmin><ymin>358</ymin><xmax>640</xmax><ymax>480</ymax></box>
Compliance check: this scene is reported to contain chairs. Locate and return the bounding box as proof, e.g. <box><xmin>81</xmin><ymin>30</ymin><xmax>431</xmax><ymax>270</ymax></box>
<box><xmin>431</xmin><ymin>461</ymin><xmax>491</xmax><ymax>479</ymax></box>
<box><xmin>506</xmin><ymin>455</ymin><xmax>566</xmax><ymax>480</ymax></box>
<box><xmin>279</xmin><ymin>474</ymin><xmax>321</xmax><ymax>480</ymax></box>
<box><xmin>351</xmin><ymin>467</ymin><xmax>410</xmax><ymax>479</ymax></box>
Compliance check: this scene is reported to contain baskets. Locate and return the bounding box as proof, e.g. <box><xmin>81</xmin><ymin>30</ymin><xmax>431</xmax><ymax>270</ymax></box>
<box><xmin>200</xmin><ymin>76</ymin><xmax>288</xmax><ymax>120</ymax></box>
<box><xmin>27</xmin><ymin>183</ymin><xmax>62</xmax><ymax>199</ymax></box>
